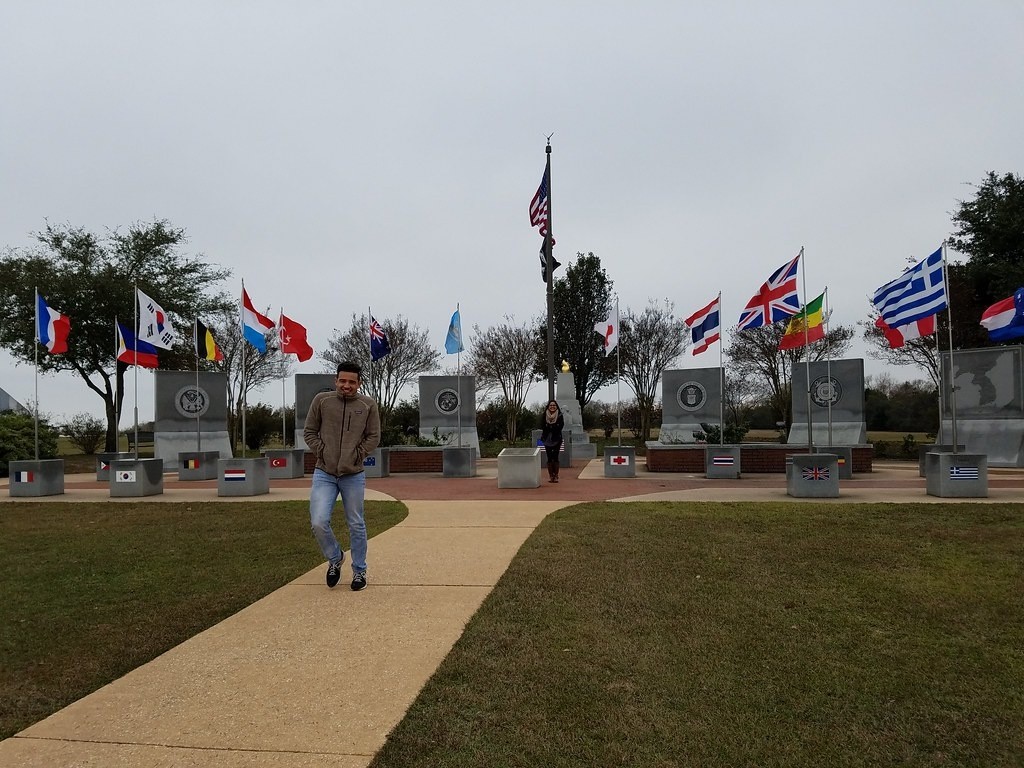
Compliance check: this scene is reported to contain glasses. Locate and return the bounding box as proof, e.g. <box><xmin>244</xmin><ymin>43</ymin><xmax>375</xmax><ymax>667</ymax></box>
<box><xmin>549</xmin><ymin>405</ymin><xmax>557</xmax><ymax>406</ymax></box>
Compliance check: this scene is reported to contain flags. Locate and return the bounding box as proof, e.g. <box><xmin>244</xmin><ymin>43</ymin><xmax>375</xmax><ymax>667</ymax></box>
<box><xmin>445</xmin><ymin>311</ymin><xmax>465</xmax><ymax>354</ymax></box>
<box><xmin>369</xmin><ymin>314</ymin><xmax>392</xmax><ymax>361</ymax></box>
<box><xmin>874</xmin><ymin>246</ymin><xmax>948</xmax><ymax>329</ymax></box>
<box><xmin>117</xmin><ymin>322</ymin><xmax>158</xmax><ymax>369</ymax></box>
<box><xmin>737</xmin><ymin>253</ymin><xmax>801</xmax><ymax>332</ymax></box>
<box><xmin>593</xmin><ymin>302</ymin><xmax>618</xmax><ymax>357</ymax></box>
<box><xmin>137</xmin><ymin>288</ymin><xmax>175</xmax><ymax>350</ymax></box>
<box><xmin>980</xmin><ymin>289</ymin><xmax>1024</xmax><ymax>339</ymax></box>
<box><xmin>684</xmin><ymin>297</ymin><xmax>720</xmax><ymax>357</ymax></box>
<box><xmin>777</xmin><ymin>293</ymin><xmax>827</xmax><ymax>349</ymax></box>
<box><xmin>240</xmin><ymin>286</ymin><xmax>275</xmax><ymax>354</ymax></box>
<box><xmin>36</xmin><ymin>294</ymin><xmax>70</xmax><ymax>355</ymax></box>
<box><xmin>530</xmin><ymin>163</ymin><xmax>561</xmax><ymax>283</ymax></box>
<box><xmin>194</xmin><ymin>317</ymin><xmax>225</xmax><ymax>360</ymax></box>
<box><xmin>279</xmin><ymin>314</ymin><xmax>313</xmax><ymax>362</ymax></box>
<box><xmin>875</xmin><ymin>313</ymin><xmax>936</xmax><ymax>348</ymax></box>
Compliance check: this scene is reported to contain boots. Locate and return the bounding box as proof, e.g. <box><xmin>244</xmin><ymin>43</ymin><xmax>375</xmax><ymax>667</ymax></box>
<box><xmin>547</xmin><ymin>461</ymin><xmax>560</xmax><ymax>482</ymax></box>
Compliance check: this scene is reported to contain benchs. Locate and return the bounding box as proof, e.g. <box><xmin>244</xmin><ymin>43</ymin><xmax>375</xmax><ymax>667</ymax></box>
<box><xmin>126</xmin><ymin>432</ymin><xmax>154</xmax><ymax>452</ymax></box>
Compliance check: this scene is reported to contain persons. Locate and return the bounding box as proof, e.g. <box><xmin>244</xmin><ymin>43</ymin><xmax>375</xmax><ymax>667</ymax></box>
<box><xmin>304</xmin><ymin>361</ymin><xmax>381</xmax><ymax>592</ymax></box>
<box><xmin>541</xmin><ymin>400</ymin><xmax>564</xmax><ymax>482</ymax></box>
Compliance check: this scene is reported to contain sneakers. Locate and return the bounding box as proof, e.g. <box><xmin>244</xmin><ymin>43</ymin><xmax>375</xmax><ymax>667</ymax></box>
<box><xmin>325</xmin><ymin>550</ymin><xmax>345</xmax><ymax>589</ymax></box>
<box><xmin>350</xmin><ymin>571</ymin><xmax>368</xmax><ymax>591</ymax></box>
